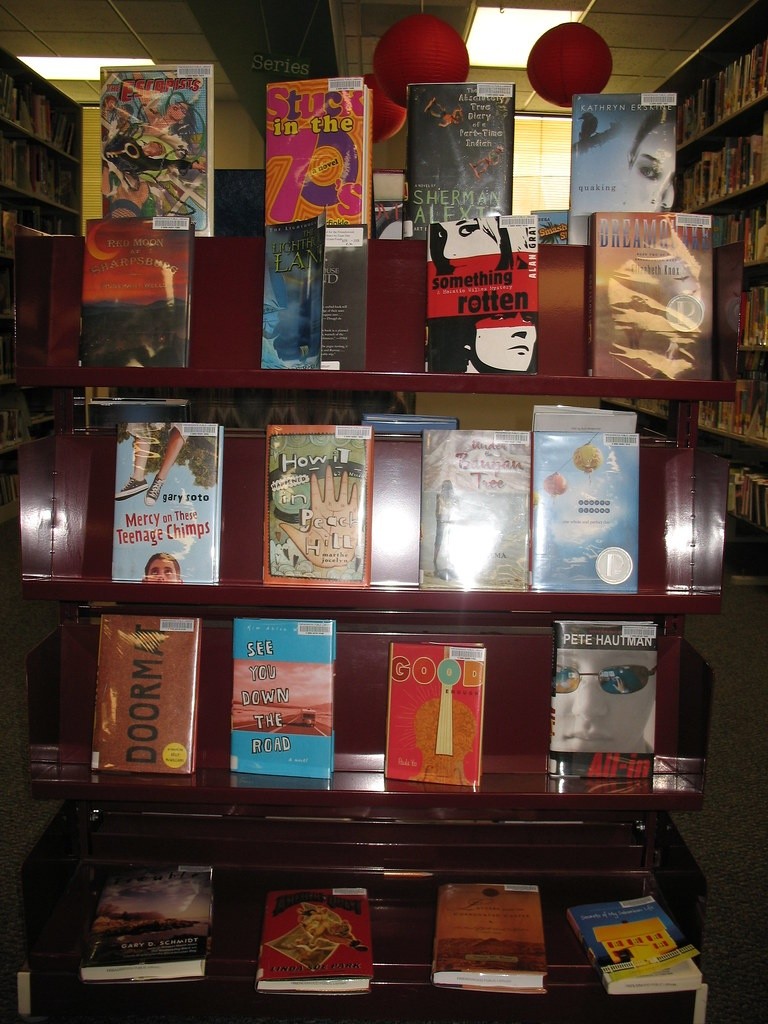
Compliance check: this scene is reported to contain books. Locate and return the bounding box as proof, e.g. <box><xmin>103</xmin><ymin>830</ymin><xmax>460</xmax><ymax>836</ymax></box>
<box><xmin>546</xmin><ymin>621</ymin><xmax>658</xmax><ymax>778</ymax></box>
<box><xmin>112</xmin><ymin>422</ymin><xmax>224</xmax><ymax>584</ymax></box>
<box><xmin>384</xmin><ymin>642</ymin><xmax>487</xmax><ymax>787</ymax></box>
<box><xmin>0</xmin><ymin>270</ymin><xmax>23</xmax><ymax>506</ymax></box>
<box><xmin>0</xmin><ymin>68</ymin><xmax>75</xmax><ymax>254</ymax></box>
<box><xmin>254</xmin><ymin>888</ymin><xmax>374</xmax><ymax>995</ymax></box>
<box><xmin>91</xmin><ymin>614</ymin><xmax>203</xmax><ymax>774</ymax></box>
<box><xmin>585</xmin><ymin>212</ymin><xmax>713</xmax><ymax>380</ymax></box>
<box><xmin>265</xmin><ymin>77</ymin><xmax>374</xmax><ymax>238</ymax></box>
<box><xmin>261</xmin><ymin>206</ymin><xmax>368</xmax><ymax>369</ymax></box>
<box><xmin>566</xmin><ymin>896</ymin><xmax>702</xmax><ymax>994</ymax></box>
<box><xmin>80</xmin><ymin>865</ymin><xmax>213</xmax><ymax>985</ymax></box>
<box><xmin>229</xmin><ymin>617</ymin><xmax>337</xmax><ymax>779</ymax></box>
<box><xmin>263</xmin><ymin>424</ymin><xmax>374</xmax><ymax>587</ymax></box>
<box><xmin>430</xmin><ymin>884</ymin><xmax>548</xmax><ymax>994</ymax></box>
<box><xmin>402</xmin><ymin>82</ymin><xmax>516</xmax><ymax>241</ymax></box>
<box><xmin>568</xmin><ymin>93</ymin><xmax>677</xmax><ymax>245</ymax></box>
<box><xmin>677</xmin><ymin>41</ymin><xmax>768</xmax><ymax>527</ymax></box>
<box><xmin>100</xmin><ymin>64</ymin><xmax>214</xmax><ymax>238</ymax></box>
<box><xmin>77</xmin><ymin>218</ymin><xmax>195</xmax><ymax>368</ymax></box>
<box><xmin>362</xmin><ymin>406</ymin><xmax>639</xmax><ymax>591</ymax></box>
<box><xmin>425</xmin><ymin>215</ymin><xmax>539</xmax><ymax>375</ymax></box>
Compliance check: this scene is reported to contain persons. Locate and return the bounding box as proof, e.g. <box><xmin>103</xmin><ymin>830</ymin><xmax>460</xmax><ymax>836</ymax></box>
<box><xmin>114</xmin><ymin>423</ymin><xmax>190</xmax><ymax>506</ymax></box>
<box><xmin>551</xmin><ymin>649</ymin><xmax>654</xmax><ymax>754</ymax></box>
<box><xmin>141</xmin><ymin>552</ymin><xmax>184</xmax><ymax>582</ymax></box>
<box><xmin>626</xmin><ymin>106</ymin><xmax>676</xmax><ymax>212</ymax></box>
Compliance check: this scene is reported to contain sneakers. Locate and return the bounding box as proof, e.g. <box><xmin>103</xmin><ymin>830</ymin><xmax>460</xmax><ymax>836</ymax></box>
<box><xmin>144</xmin><ymin>475</ymin><xmax>165</xmax><ymax>506</ymax></box>
<box><xmin>115</xmin><ymin>476</ymin><xmax>148</xmax><ymax>501</ymax></box>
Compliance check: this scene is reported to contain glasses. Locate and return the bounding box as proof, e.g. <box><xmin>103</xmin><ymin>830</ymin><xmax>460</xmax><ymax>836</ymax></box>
<box><xmin>556</xmin><ymin>664</ymin><xmax>657</xmax><ymax>694</ymax></box>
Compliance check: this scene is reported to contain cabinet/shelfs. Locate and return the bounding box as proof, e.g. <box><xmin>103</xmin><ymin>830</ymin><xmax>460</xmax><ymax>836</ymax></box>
<box><xmin>0</xmin><ymin>46</ymin><xmax>85</xmax><ymax>524</ymax></box>
<box><xmin>601</xmin><ymin>0</ymin><xmax>768</xmax><ymax>570</ymax></box>
<box><xmin>11</xmin><ymin>236</ymin><xmax>744</xmax><ymax>1024</ymax></box>
